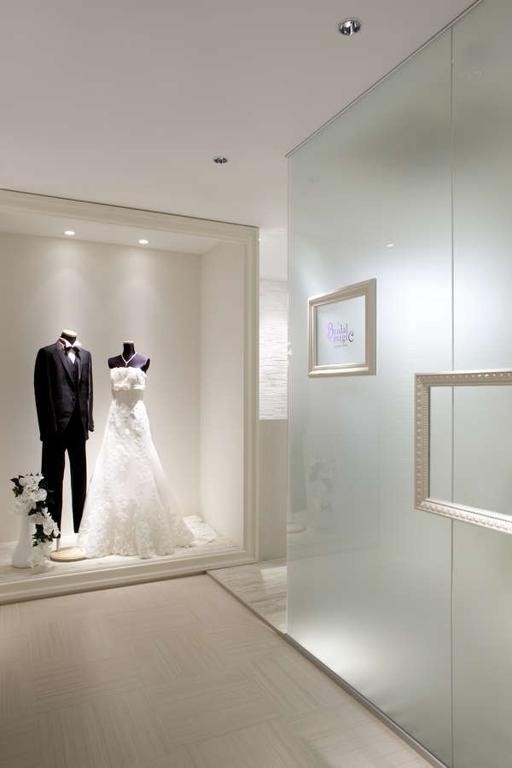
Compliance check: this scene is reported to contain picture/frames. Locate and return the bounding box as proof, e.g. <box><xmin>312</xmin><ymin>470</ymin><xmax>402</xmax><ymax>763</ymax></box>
<box><xmin>309</xmin><ymin>278</ymin><xmax>377</xmax><ymax>375</ymax></box>
<box><xmin>414</xmin><ymin>372</ymin><xmax>512</xmax><ymax>533</ymax></box>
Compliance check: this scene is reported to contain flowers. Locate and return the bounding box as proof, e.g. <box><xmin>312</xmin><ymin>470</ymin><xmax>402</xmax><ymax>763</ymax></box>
<box><xmin>9</xmin><ymin>472</ymin><xmax>60</xmax><ymax>576</ymax></box>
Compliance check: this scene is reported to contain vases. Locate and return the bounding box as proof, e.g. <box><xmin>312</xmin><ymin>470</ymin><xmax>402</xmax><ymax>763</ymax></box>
<box><xmin>11</xmin><ymin>510</ymin><xmax>35</xmax><ymax>568</ymax></box>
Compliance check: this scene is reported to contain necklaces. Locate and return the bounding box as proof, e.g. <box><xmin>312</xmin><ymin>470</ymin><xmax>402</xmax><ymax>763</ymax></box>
<box><xmin>120</xmin><ymin>351</ymin><xmax>138</xmax><ymax>367</ymax></box>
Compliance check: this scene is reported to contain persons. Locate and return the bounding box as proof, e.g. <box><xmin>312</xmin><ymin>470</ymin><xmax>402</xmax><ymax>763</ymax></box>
<box><xmin>73</xmin><ymin>340</ymin><xmax>198</xmax><ymax>561</ymax></box>
<box><xmin>31</xmin><ymin>329</ymin><xmax>95</xmax><ymax>554</ymax></box>
